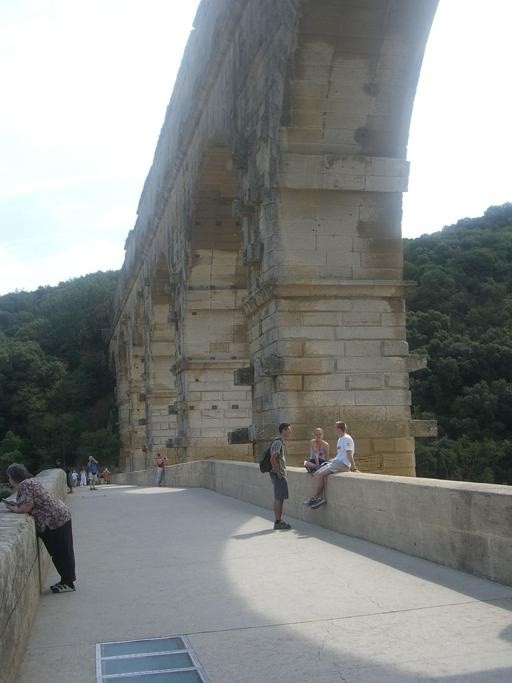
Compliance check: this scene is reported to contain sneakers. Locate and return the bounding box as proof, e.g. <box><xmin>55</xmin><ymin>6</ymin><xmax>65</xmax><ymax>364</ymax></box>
<box><xmin>273</xmin><ymin>518</ymin><xmax>291</xmax><ymax>529</ymax></box>
<box><xmin>307</xmin><ymin>494</ymin><xmax>326</xmax><ymax>510</ymax></box>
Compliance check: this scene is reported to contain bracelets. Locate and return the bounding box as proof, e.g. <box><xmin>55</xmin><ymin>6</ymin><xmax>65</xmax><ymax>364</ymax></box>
<box><xmin>353</xmin><ymin>467</ymin><xmax>358</xmax><ymax>471</ymax></box>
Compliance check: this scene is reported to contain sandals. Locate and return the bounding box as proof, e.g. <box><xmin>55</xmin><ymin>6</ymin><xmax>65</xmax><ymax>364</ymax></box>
<box><xmin>48</xmin><ymin>581</ymin><xmax>74</xmax><ymax>593</ymax></box>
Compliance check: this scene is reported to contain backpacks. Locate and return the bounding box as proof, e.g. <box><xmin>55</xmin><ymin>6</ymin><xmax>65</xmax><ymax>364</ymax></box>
<box><xmin>259</xmin><ymin>447</ymin><xmax>272</xmax><ymax>472</ymax></box>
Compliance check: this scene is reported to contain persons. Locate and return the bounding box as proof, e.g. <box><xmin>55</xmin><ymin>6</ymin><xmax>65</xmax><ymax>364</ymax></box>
<box><xmin>156</xmin><ymin>452</ymin><xmax>168</xmax><ymax>486</ymax></box>
<box><xmin>302</xmin><ymin>426</ymin><xmax>330</xmax><ymax>473</ymax></box>
<box><xmin>266</xmin><ymin>421</ymin><xmax>292</xmax><ymax>530</ymax></box>
<box><xmin>53</xmin><ymin>454</ymin><xmax>111</xmax><ymax>493</ymax></box>
<box><xmin>1</xmin><ymin>461</ymin><xmax>79</xmax><ymax>595</ymax></box>
<box><xmin>301</xmin><ymin>419</ymin><xmax>362</xmax><ymax>510</ymax></box>
